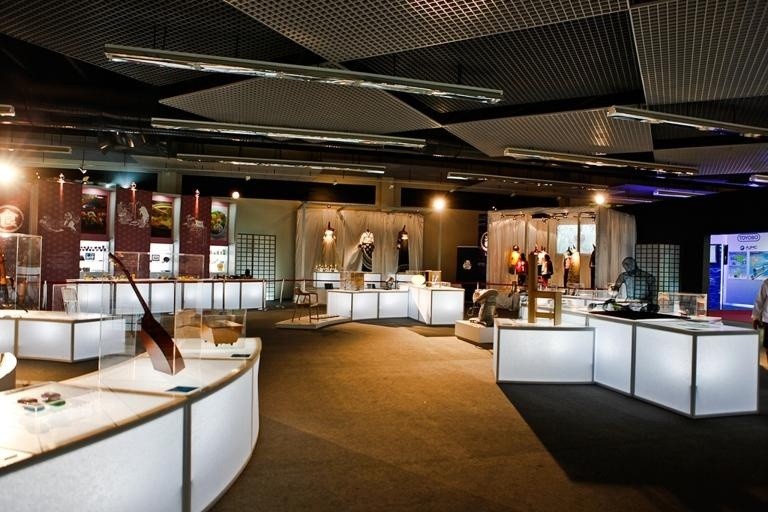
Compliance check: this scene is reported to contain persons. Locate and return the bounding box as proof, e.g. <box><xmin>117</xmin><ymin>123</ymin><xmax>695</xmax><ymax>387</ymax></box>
<box><xmin>615</xmin><ymin>256</ymin><xmax>658</xmax><ymax>304</ymax></box>
<box><xmin>240</xmin><ymin>269</ymin><xmax>253</xmax><ymax>279</ymax></box>
<box><xmin>752</xmin><ymin>278</ymin><xmax>768</xmax><ymax>372</ymax></box>
<box><xmin>358</xmin><ymin>229</ymin><xmax>374</xmax><ymax>272</ymax></box>
<box><xmin>508</xmin><ymin>244</ymin><xmax>521</xmax><ymax>275</ymax></box>
<box><xmin>541</xmin><ymin>254</ymin><xmax>553</xmax><ymax>290</ymax></box>
<box><xmin>563</xmin><ymin>251</ymin><xmax>572</xmax><ymax>287</ymax></box>
<box><xmin>322</xmin><ymin>229</ymin><xmax>336</xmax><ymax>268</ymax></box>
<box><xmin>396</xmin><ymin>230</ymin><xmax>409</xmax><ymax>272</ymax></box>
<box><xmin>516</xmin><ymin>252</ymin><xmax>529</xmax><ymax>285</ymax></box>
<box><xmin>534</xmin><ymin>245</ymin><xmax>547</xmax><ymax>283</ymax></box>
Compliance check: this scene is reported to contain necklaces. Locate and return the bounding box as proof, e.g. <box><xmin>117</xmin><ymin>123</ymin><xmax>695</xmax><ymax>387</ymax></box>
<box><xmin>365</xmin><ymin>232</ymin><xmax>371</xmax><ymax>238</ymax></box>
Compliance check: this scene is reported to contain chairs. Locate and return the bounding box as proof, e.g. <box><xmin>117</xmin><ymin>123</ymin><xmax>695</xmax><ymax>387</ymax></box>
<box><xmin>293</xmin><ymin>287</ymin><xmax>321</xmax><ymax>322</ymax></box>
